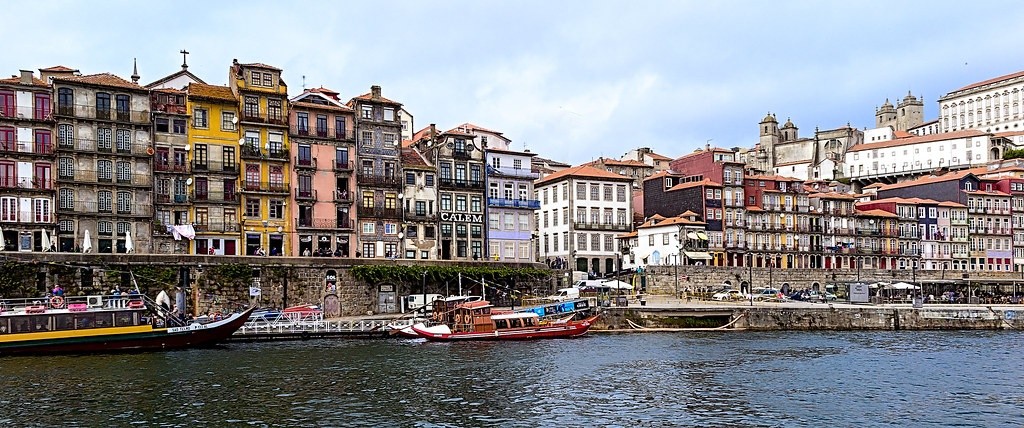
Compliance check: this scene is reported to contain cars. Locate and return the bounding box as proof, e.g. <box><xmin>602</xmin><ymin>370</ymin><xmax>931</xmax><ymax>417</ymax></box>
<box><xmin>744</xmin><ymin>286</ymin><xmax>785</xmax><ymax>302</ymax></box>
<box><xmin>787</xmin><ymin>288</ymin><xmax>837</xmax><ymax>303</ymax></box>
<box><xmin>711</xmin><ymin>289</ymin><xmax>743</xmax><ymax>302</ymax></box>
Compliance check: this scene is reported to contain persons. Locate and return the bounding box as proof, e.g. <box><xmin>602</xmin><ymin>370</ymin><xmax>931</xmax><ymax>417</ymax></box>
<box><xmin>50</xmin><ymin>242</ymin><xmax>80</xmax><ymax>253</ymax></box>
<box><xmin>473</xmin><ymin>253</ymin><xmax>478</xmax><ymax>260</ymax></box>
<box><xmin>873</xmin><ymin>287</ymin><xmax>1024</xmax><ymax>303</ymax></box>
<box><xmin>788</xmin><ymin>286</ymin><xmax>828</xmax><ymax>303</ymax></box>
<box><xmin>684</xmin><ymin>285</ymin><xmax>712</xmax><ymax>301</ymax></box>
<box><xmin>302</xmin><ymin>248</ymin><xmax>312</xmax><ymax>257</ymax></box>
<box><xmin>272</xmin><ymin>247</ymin><xmax>277</xmax><ymax>255</ymax></box>
<box><xmin>313</xmin><ymin>245</ymin><xmax>333</xmax><ymax>258</ymax></box>
<box><xmin>495</xmin><ymin>254</ymin><xmax>500</xmax><ymax>261</ymax></box>
<box><xmin>541</xmin><ymin>256</ymin><xmax>568</xmax><ymax>269</ymax></box>
<box><xmin>47</xmin><ymin>283</ymin><xmax>140</xmax><ymax>302</ymax></box>
<box><xmin>355</xmin><ymin>250</ymin><xmax>362</xmax><ymax>258</ymax></box>
<box><xmin>254</xmin><ymin>246</ymin><xmax>266</xmax><ymax>256</ymax></box>
<box><xmin>536</xmin><ymin>258</ymin><xmax>540</xmax><ymax>262</ymax></box>
<box><xmin>209</xmin><ymin>246</ymin><xmax>215</xmax><ymax>254</ymax></box>
<box><xmin>334</xmin><ymin>249</ymin><xmax>341</xmax><ymax>257</ymax></box>
<box><xmin>777</xmin><ymin>290</ymin><xmax>785</xmax><ymax>302</ymax></box>
<box><xmin>386</xmin><ymin>251</ymin><xmax>390</xmax><ymax>257</ymax></box>
<box><xmin>633</xmin><ymin>287</ymin><xmax>640</xmax><ymax>302</ymax></box>
<box><xmin>159</xmin><ymin>300</ymin><xmax>194</xmax><ymax>326</ymax></box>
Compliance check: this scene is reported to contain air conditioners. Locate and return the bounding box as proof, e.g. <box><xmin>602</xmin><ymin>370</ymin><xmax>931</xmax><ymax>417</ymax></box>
<box><xmin>87</xmin><ymin>295</ymin><xmax>104</xmax><ymax>307</ymax></box>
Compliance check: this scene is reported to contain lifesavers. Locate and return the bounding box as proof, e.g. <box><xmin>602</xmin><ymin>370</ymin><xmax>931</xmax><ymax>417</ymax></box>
<box><xmin>208</xmin><ymin>312</ymin><xmax>223</xmax><ymax>321</ymax></box>
<box><xmin>456</xmin><ymin>314</ymin><xmax>469</xmax><ymax>324</ymax></box>
<box><xmin>301</xmin><ymin>332</ymin><xmax>310</xmax><ymax>341</ymax></box>
<box><xmin>129</xmin><ymin>301</ymin><xmax>143</xmax><ymax>308</ymax></box>
<box><xmin>433</xmin><ymin>312</ymin><xmax>442</xmax><ymax>321</ymax></box>
<box><xmin>51</xmin><ymin>295</ymin><xmax>63</xmax><ymax>307</ymax></box>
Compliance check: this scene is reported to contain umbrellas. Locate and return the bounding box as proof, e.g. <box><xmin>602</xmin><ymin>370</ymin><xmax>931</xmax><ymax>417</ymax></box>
<box><xmin>83</xmin><ymin>229</ymin><xmax>92</xmax><ymax>253</ymax></box>
<box><xmin>41</xmin><ymin>228</ymin><xmax>52</xmax><ymax>252</ymax></box>
<box><xmin>0</xmin><ymin>226</ymin><xmax>6</xmax><ymax>252</ymax></box>
<box><xmin>126</xmin><ymin>230</ymin><xmax>134</xmax><ymax>254</ymax></box>
<box><xmin>601</xmin><ymin>278</ymin><xmax>634</xmax><ymax>289</ymax></box>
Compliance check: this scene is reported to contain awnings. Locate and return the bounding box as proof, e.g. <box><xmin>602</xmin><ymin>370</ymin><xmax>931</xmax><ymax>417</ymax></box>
<box><xmin>683</xmin><ymin>251</ymin><xmax>713</xmax><ymax>261</ymax></box>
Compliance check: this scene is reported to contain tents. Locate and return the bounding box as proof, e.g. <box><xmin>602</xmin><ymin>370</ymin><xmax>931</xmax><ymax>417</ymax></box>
<box><xmin>869</xmin><ymin>279</ymin><xmax>921</xmax><ymax>300</ymax></box>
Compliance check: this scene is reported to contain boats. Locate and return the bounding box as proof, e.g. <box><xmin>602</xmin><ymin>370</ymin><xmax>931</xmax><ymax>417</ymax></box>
<box><xmin>387</xmin><ymin>274</ymin><xmax>604</xmax><ymax>342</ymax></box>
<box><xmin>0</xmin><ymin>265</ymin><xmax>258</xmax><ymax>355</ymax></box>
